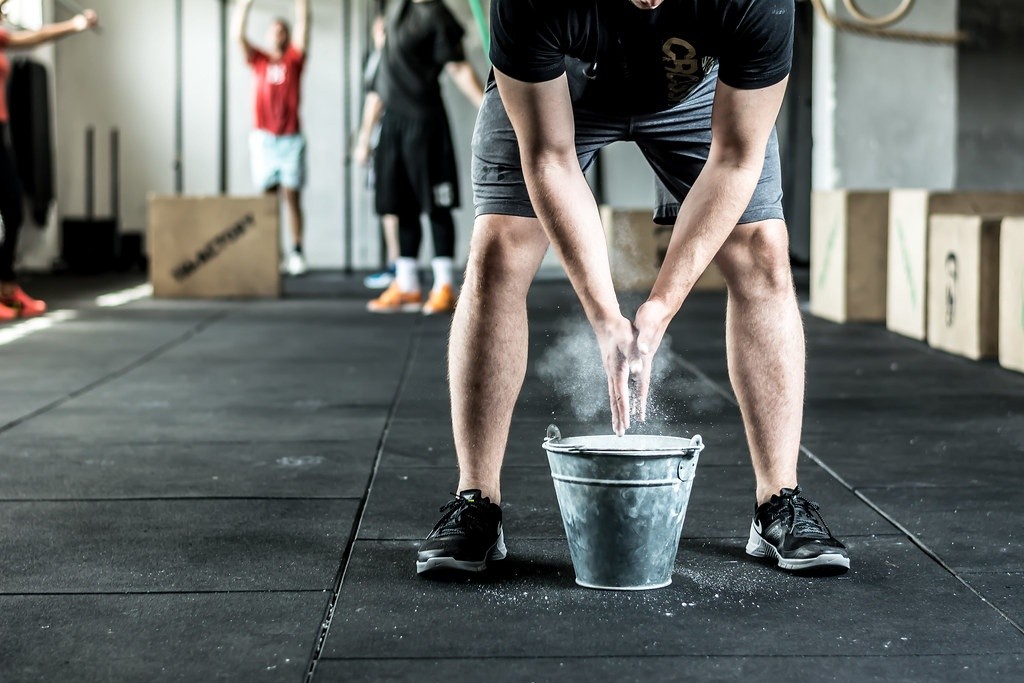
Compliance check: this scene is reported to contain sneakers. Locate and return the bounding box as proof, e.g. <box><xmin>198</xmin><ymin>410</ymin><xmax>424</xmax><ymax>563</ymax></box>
<box><xmin>417</xmin><ymin>487</ymin><xmax>506</xmax><ymax>574</ymax></box>
<box><xmin>746</xmin><ymin>486</ymin><xmax>852</xmax><ymax>571</ymax></box>
<box><xmin>367</xmin><ymin>282</ymin><xmax>423</xmax><ymax>313</ymax></box>
<box><xmin>0</xmin><ymin>288</ymin><xmax>46</xmax><ymax>322</ymax></box>
<box><xmin>421</xmin><ymin>285</ymin><xmax>458</xmax><ymax>317</ymax></box>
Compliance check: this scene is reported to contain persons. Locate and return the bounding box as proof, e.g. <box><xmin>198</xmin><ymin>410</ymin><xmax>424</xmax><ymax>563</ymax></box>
<box><xmin>0</xmin><ymin>0</ymin><xmax>99</xmax><ymax>325</ymax></box>
<box><xmin>414</xmin><ymin>0</ymin><xmax>850</xmax><ymax>577</ymax></box>
<box><xmin>351</xmin><ymin>0</ymin><xmax>485</xmax><ymax>315</ymax></box>
<box><xmin>355</xmin><ymin>12</ymin><xmax>400</xmax><ymax>288</ymax></box>
<box><xmin>235</xmin><ymin>0</ymin><xmax>311</xmax><ymax>277</ymax></box>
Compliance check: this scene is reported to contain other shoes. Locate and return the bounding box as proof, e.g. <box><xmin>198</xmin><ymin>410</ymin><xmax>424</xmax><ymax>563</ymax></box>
<box><xmin>364</xmin><ymin>271</ymin><xmax>397</xmax><ymax>290</ymax></box>
<box><xmin>288</xmin><ymin>255</ymin><xmax>306</xmax><ymax>275</ymax></box>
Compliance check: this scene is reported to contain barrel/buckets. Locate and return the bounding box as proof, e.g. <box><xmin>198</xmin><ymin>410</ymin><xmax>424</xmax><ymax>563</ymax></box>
<box><xmin>542</xmin><ymin>424</ymin><xmax>706</xmax><ymax>590</ymax></box>
